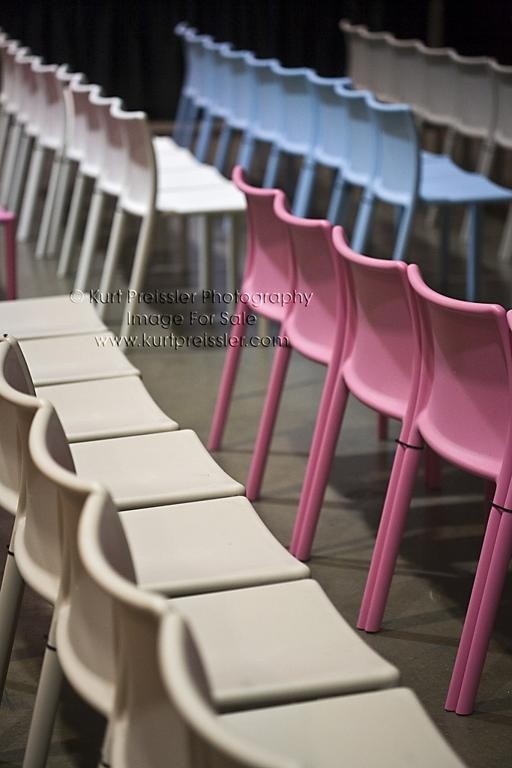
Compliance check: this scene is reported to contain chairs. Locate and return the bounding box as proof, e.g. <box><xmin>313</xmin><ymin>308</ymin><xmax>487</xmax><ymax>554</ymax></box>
<box><xmin>0</xmin><ymin>19</ymin><xmax>512</xmax><ymax>768</ymax></box>
